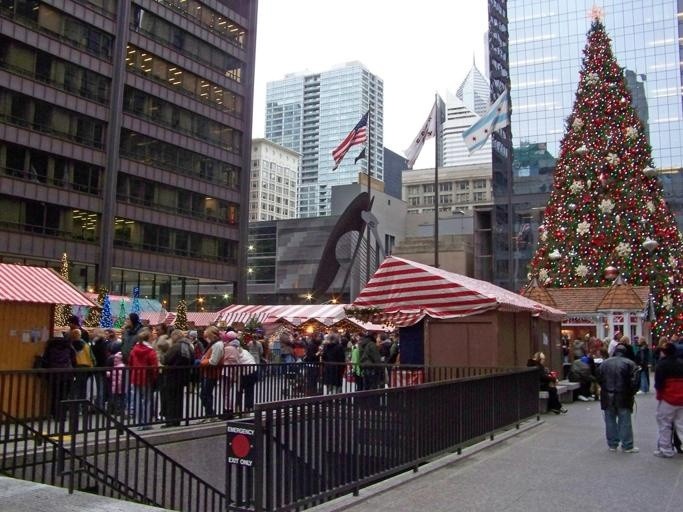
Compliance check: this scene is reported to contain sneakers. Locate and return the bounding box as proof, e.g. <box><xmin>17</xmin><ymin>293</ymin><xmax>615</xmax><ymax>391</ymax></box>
<box><xmin>623</xmin><ymin>447</ymin><xmax>639</xmax><ymax>453</ymax></box>
<box><xmin>654</xmin><ymin>451</ymin><xmax>666</xmax><ymax>457</ymax></box>
<box><xmin>552</xmin><ymin>406</ymin><xmax>567</xmax><ymax>413</ymax></box>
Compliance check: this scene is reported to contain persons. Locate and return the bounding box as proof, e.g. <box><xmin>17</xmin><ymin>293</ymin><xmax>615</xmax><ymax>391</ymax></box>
<box><xmin>30</xmin><ymin>313</ymin><xmax>399</xmax><ymax>431</ymax></box>
<box><xmin>526</xmin><ymin>329</ymin><xmax>682</xmax><ymax>458</ymax></box>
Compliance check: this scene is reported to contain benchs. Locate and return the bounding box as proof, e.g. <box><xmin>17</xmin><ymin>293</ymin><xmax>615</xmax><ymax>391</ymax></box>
<box><xmin>560</xmin><ymin>382</ymin><xmax>580</xmax><ymax>402</ymax></box>
<box><xmin>538</xmin><ymin>385</ymin><xmax>567</xmax><ymax>415</ymax></box>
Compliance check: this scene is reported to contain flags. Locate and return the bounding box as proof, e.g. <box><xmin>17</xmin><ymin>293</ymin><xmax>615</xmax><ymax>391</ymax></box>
<box><xmin>404</xmin><ymin>101</ymin><xmax>436</xmax><ymax>169</ymax></box>
<box><xmin>461</xmin><ymin>88</ymin><xmax>510</xmax><ymax>155</ymax></box>
<box><xmin>332</xmin><ymin>110</ymin><xmax>368</xmax><ymax>166</ymax></box>
